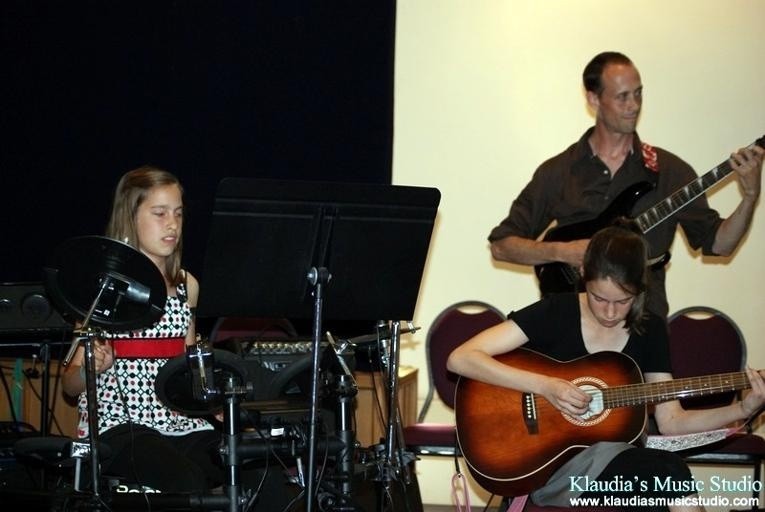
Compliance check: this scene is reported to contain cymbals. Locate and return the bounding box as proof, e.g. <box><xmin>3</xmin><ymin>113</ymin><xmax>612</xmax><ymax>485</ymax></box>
<box><xmin>50</xmin><ymin>235</ymin><xmax>167</xmax><ymax>331</ymax></box>
<box><xmin>155</xmin><ymin>350</ymin><xmax>246</xmax><ymax>417</ymax></box>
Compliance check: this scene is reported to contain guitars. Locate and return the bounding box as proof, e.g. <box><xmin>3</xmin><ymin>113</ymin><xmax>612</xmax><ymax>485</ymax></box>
<box><xmin>535</xmin><ymin>136</ymin><xmax>765</xmax><ymax>295</ymax></box>
<box><xmin>455</xmin><ymin>347</ymin><xmax>764</xmax><ymax>496</ymax></box>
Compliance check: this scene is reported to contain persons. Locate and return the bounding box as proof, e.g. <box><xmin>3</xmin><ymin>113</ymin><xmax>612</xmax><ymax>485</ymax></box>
<box><xmin>488</xmin><ymin>52</ymin><xmax>765</xmax><ymax>318</ymax></box>
<box><xmin>446</xmin><ymin>228</ymin><xmax>764</xmax><ymax>512</ymax></box>
<box><xmin>60</xmin><ymin>166</ymin><xmax>291</xmax><ymax>512</ymax></box>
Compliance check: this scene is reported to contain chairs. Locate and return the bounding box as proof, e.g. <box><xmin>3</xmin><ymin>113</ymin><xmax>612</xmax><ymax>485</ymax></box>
<box><xmin>636</xmin><ymin>306</ymin><xmax>764</xmax><ymax>510</ymax></box>
<box><xmin>403</xmin><ymin>300</ymin><xmax>512</xmax><ymax>457</ymax></box>
